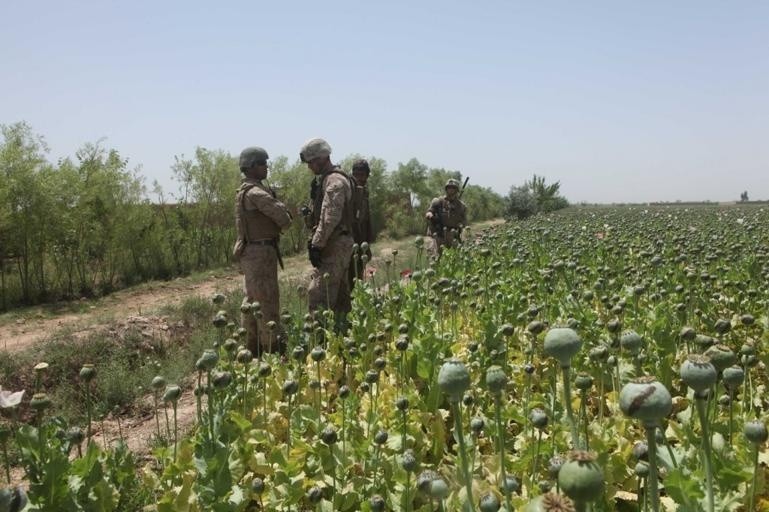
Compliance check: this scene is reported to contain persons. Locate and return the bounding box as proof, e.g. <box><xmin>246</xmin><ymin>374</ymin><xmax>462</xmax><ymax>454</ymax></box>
<box><xmin>299</xmin><ymin>138</ymin><xmax>359</xmax><ymax>348</ymax></box>
<box><xmin>232</xmin><ymin>146</ymin><xmax>293</xmax><ymax>359</ymax></box>
<box><xmin>351</xmin><ymin>157</ymin><xmax>372</xmax><ymax>281</ymax></box>
<box><xmin>422</xmin><ymin>177</ymin><xmax>470</xmax><ymax>267</ymax></box>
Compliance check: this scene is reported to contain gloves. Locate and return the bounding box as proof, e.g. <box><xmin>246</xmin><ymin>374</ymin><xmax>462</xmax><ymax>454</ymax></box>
<box><xmin>309</xmin><ymin>246</ymin><xmax>320</xmax><ymax>268</ymax></box>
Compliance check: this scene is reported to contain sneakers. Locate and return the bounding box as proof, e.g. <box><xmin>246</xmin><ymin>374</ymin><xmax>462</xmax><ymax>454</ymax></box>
<box><xmin>247</xmin><ymin>338</ymin><xmax>288</xmax><ymax>357</ymax></box>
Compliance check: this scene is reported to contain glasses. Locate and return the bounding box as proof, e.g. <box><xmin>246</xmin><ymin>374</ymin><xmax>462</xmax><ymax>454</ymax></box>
<box><xmin>254</xmin><ymin>160</ymin><xmax>267</xmax><ymax>166</ymax></box>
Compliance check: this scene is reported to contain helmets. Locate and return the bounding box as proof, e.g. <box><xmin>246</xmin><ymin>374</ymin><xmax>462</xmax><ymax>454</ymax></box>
<box><xmin>239</xmin><ymin>146</ymin><xmax>269</xmax><ymax>171</ymax></box>
<box><xmin>352</xmin><ymin>159</ymin><xmax>370</xmax><ymax>179</ymax></box>
<box><xmin>300</xmin><ymin>138</ymin><xmax>331</xmax><ymax>162</ymax></box>
<box><xmin>444</xmin><ymin>178</ymin><xmax>459</xmax><ymax>194</ymax></box>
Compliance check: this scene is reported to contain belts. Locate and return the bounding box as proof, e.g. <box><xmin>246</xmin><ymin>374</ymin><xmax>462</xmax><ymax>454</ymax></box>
<box><xmin>245</xmin><ymin>240</ymin><xmax>278</xmax><ymax>246</ymax></box>
